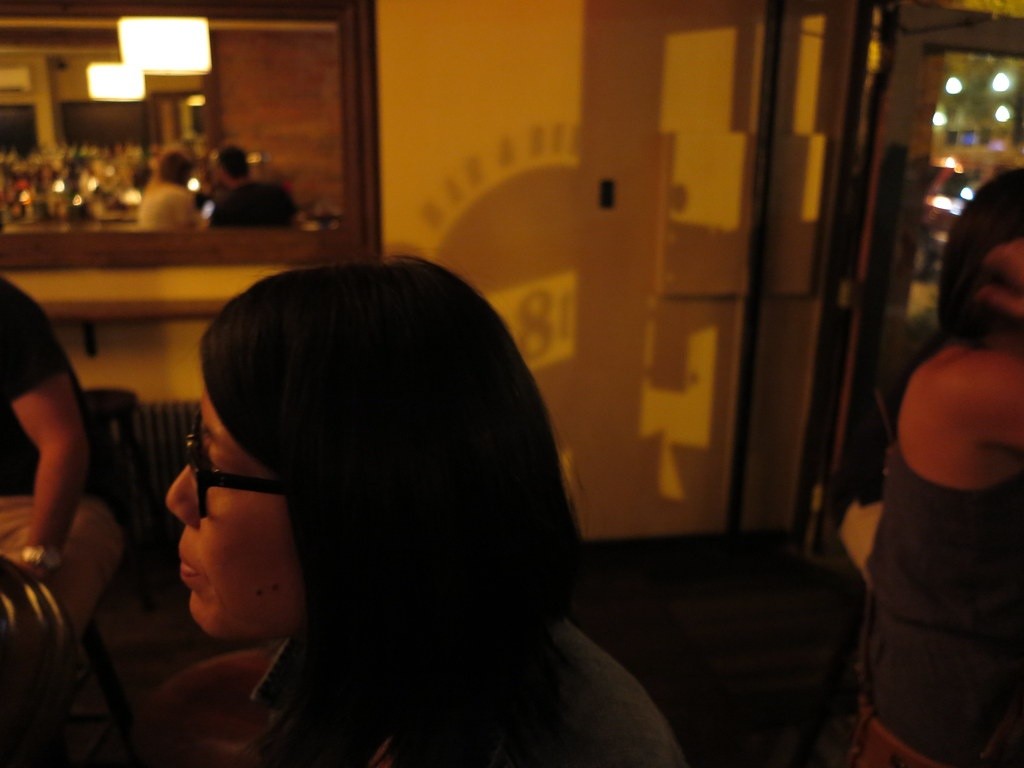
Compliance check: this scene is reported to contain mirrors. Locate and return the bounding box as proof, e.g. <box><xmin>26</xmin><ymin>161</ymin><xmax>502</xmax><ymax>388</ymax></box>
<box><xmin>0</xmin><ymin>0</ymin><xmax>385</xmax><ymax>270</ymax></box>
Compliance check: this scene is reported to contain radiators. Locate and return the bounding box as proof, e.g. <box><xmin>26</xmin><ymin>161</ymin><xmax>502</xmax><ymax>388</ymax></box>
<box><xmin>109</xmin><ymin>399</ymin><xmax>198</xmax><ymax>551</ymax></box>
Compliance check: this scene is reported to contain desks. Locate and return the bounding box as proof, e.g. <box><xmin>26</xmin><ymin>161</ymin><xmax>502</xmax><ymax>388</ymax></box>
<box><xmin>0</xmin><ymin>555</ymin><xmax>77</xmax><ymax>768</ymax></box>
<box><xmin>38</xmin><ymin>299</ymin><xmax>225</xmax><ymax>360</ymax></box>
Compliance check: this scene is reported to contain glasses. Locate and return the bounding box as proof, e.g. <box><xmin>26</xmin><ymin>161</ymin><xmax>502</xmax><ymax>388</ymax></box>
<box><xmin>185</xmin><ymin>408</ymin><xmax>287</xmax><ymax>518</ymax></box>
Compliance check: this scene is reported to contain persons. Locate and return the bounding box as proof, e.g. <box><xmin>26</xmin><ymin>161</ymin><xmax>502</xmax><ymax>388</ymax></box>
<box><xmin>136</xmin><ymin>135</ymin><xmax>297</xmax><ymax>228</ymax></box>
<box><xmin>844</xmin><ymin>167</ymin><xmax>1024</xmax><ymax>767</ymax></box>
<box><xmin>0</xmin><ymin>277</ymin><xmax>92</xmax><ymax>592</ymax></box>
<box><xmin>162</xmin><ymin>254</ymin><xmax>693</xmax><ymax>768</ymax></box>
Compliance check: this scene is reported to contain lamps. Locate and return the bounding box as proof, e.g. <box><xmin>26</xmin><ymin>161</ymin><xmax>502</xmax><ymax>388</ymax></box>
<box><xmin>116</xmin><ymin>16</ymin><xmax>211</xmax><ymax>75</ymax></box>
<box><xmin>85</xmin><ymin>62</ymin><xmax>147</xmax><ymax>102</ymax></box>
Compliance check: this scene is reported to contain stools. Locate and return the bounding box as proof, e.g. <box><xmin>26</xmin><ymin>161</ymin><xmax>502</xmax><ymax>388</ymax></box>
<box><xmin>83</xmin><ymin>388</ymin><xmax>173</xmax><ymax>613</ymax></box>
<box><xmin>844</xmin><ymin>700</ymin><xmax>956</xmax><ymax>768</ymax></box>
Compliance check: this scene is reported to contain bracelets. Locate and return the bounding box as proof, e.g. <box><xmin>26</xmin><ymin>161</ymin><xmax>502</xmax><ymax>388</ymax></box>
<box><xmin>22</xmin><ymin>544</ymin><xmax>64</xmax><ymax>572</ymax></box>
